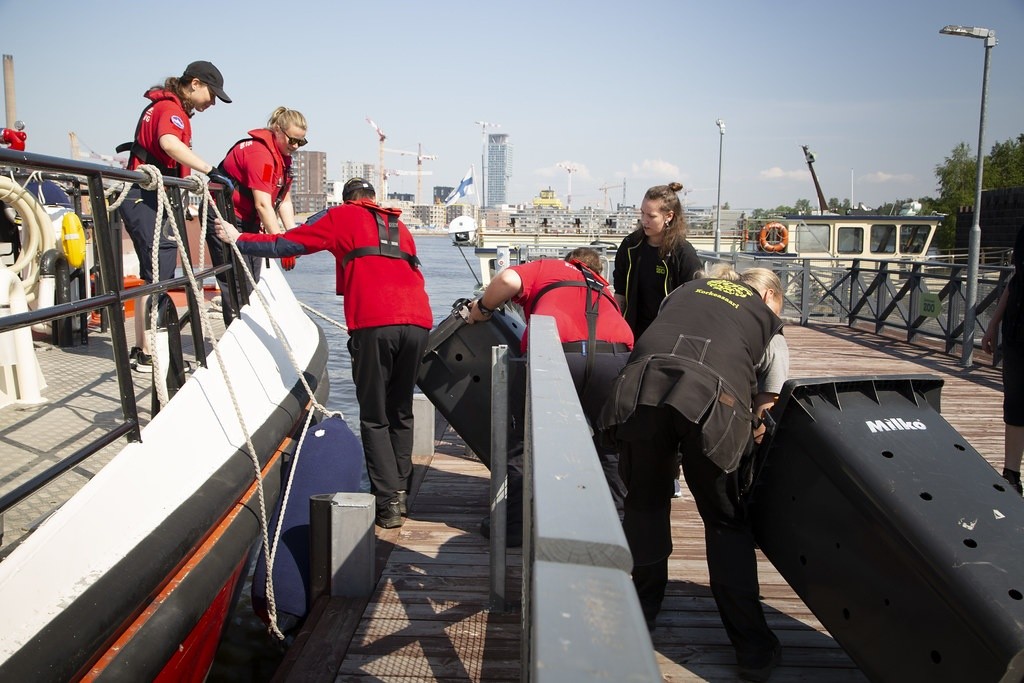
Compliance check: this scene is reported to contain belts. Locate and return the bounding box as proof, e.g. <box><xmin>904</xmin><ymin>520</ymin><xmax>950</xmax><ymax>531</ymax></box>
<box><xmin>562</xmin><ymin>341</ymin><xmax>630</xmax><ymax>353</ymax></box>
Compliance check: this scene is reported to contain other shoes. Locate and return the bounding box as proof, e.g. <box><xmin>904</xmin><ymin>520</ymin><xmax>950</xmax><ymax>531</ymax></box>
<box><xmin>737</xmin><ymin>631</ymin><xmax>783</xmax><ymax>683</ymax></box>
<box><xmin>672</xmin><ymin>479</ymin><xmax>682</xmax><ymax>497</ymax></box>
<box><xmin>375</xmin><ymin>491</ymin><xmax>410</xmax><ymax>528</ymax></box>
<box><xmin>479</xmin><ymin>514</ymin><xmax>523</xmax><ymax>546</ymax></box>
<box><xmin>130</xmin><ymin>345</ymin><xmax>190</xmax><ymax>373</ymax></box>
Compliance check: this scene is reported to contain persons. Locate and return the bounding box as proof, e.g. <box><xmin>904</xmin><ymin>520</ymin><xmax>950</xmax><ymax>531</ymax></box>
<box><xmin>116</xmin><ymin>61</ymin><xmax>233</xmax><ymax>373</ymax></box>
<box><xmin>604</xmin><ymin>262</ymin><xmax>789</xmax><ymax>683</ymax></box>
<box><xmin>981</xmin><ymin>220</ymin><xmax>1024</xmax><ymax>494</ymax></box>
<box><xmin>467</xmin><ymin>248</ymin><xmax>636</xmax><ymax>522</ymax></box>
<box><xmin>612</xmin><ymin>184</ymin><xmax>705</xmax><ymax>498</ymax></box>
<box><xmin>197</xmin><ymin>107</ymin><xmax>307</xmax><ymax>329</ymax></box>
<box><xmin>215</xmin><ymin>175</ymin><xmax>433</xmax><ymax>529</ymax></box>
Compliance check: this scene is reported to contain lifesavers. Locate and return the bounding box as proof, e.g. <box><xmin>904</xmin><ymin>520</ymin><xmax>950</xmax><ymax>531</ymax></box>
<box><xmin>60</xmin><ymin>212</ymin><xmax>87</xmax><ymax>269</ymax></box>
<box><xmin>759</xmin><ymin>222</ymin><xmax>788</xmax><ymax>252</ymax></box>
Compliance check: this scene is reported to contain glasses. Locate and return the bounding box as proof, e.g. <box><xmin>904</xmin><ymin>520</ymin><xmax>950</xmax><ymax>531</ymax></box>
<box><xmin>281</xmin><ymin>130</ymin><xmax>308</xmax><ymax>146</ymax></box>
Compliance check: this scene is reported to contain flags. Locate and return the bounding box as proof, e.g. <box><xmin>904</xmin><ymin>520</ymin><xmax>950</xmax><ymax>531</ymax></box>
<box><xmin>443</xmin><ymin>162</ymin><xmax>482</xmax><ymax>207</ymax></box>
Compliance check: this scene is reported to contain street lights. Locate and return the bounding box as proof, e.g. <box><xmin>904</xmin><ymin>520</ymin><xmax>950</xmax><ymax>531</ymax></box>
<box><xmin>940</xmin><ymin>26</ymin><xmax>999</xmax><ymax>368</ymax></box>
<box><xmin>715</xmin><ymin>119</ymin><xmax>726</xmax><ymax>252</ymax></box>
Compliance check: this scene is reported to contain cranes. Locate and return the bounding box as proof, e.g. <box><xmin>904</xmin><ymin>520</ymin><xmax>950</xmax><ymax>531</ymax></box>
<box><xmin>385</xmin><ymin>143</ymin><xmax>436</xmax><ymax>204</ymax></box>
<box><xmin>599</xmin><ymin>182</ymin><xmax>622</xmax><ymax>208</ymax></box>
<box><xmin>365</xmin><ymin>116</ymin><xmax>388</xmax><ymax>202</ymax></box>
<box><xmin>475</xmin><ymin>121</ymin><xmax>501</xmax><ymax>152</ymax></box>
<box><xmin>68</xmin><ymin>131</ymin><xmax>128</xmax><ymax>163</ymax></box>
<box><xmin>567</xmin><ymin>168</ymin><xmax>577</xmax><ymax>204</ymax></box>
<box><xmin>383</xmin><ymin>170</ymin><xmax>432</xmax><ymax>202</ymax></box>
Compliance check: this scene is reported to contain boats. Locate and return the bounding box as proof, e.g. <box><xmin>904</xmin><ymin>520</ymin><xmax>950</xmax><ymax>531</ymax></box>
<box><xmin>448</xmin><ymin>216</ymin><xmax>478</xmax><ymax>245</ymax></box>
<box><xmin>740</xmin><ymin>214</ymin><xmax>949</xmax><ymax>284</ymax></box>
<box><xmin>0</xmin><ymin>120</ymin><xmax>331</xmax><ymax>683</ymax></box>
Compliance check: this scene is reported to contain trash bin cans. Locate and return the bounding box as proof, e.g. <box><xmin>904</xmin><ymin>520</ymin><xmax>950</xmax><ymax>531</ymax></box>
<box><xmin>414</xmin><ymin>290</ymin><xmax>525</xmax><ymax>546</ymax></box>
<box><xmin>737</xmin><ymin>376</ymin><xmax>1024</xmax><ymax>683</ymax></box>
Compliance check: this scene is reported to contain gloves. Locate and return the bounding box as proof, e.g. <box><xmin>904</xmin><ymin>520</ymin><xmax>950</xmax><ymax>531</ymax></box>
<box><xmin>282</xmin><ymin>255</ymin><xmax>302</xmax><ymax>271</ymax></box>
<box><xmin>207</xmin><ymin>166</ymin><xmax>234</xmax><ymax>199</ymax></box>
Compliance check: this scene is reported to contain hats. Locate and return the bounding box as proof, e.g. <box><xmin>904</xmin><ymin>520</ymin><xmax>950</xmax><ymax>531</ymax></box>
<box><xmin>183</xmin><ymin>61</ymin><xmax>232</xmax><ymax>104</ymax></box>
<box><xmin>343</xmin><ymin>177</ymin><xmax>375</xmax><ymax>199</ymax></box>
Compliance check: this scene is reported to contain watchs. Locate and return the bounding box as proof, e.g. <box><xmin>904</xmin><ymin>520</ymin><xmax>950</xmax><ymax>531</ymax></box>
<box><xmin>477</xmin><ymin>298</ymin><xmax>496</xmax><ymax>318</ymax></box>
